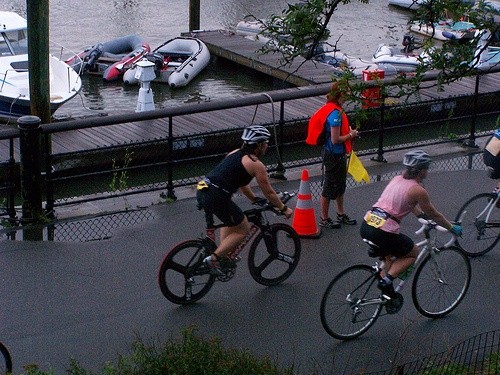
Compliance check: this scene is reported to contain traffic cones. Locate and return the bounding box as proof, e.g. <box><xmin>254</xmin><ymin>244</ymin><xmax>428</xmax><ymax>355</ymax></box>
<box><xmin>286</xmin><ymin>168</ymin><xmax>323</xmax><ymax>240</ymax></box>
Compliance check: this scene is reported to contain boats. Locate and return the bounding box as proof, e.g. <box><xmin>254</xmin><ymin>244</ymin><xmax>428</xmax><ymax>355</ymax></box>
<box><xmin>235</xmin><ymin>0</ymin><xmax>500</xmax><ymax>80</ymax></box>
<box><xmin>64</xmin><ymin>33</ymin><xmax>151</xmax><ymax>82</ymax></box>
<box><xmin>0</xmin><ymin>11</ymin><xmax>84</xmax><ymax>124</ymax></box>
<box><xmin>123</xmin><ymin>35</ymin><xmax>210</xmax><ymax>90</ymax></box>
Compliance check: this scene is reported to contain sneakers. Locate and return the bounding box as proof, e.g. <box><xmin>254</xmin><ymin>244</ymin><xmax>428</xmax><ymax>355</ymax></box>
<box><xmin>492</xmin><ymin>186</ymin><xmax>500</xmax><ymax>193</ymax></box>
<box><xmin>202</xmin><ymin>252</ymin><xmax>226</xmax><ymax>276</ymax></box>
<box><xmin>318</xmin><ymin>216</ymin><xmax>341</xmax><ymax>229</ymax></box>
<box><xmin>234</xmin><ymin>256</ymin><xmax>241</xmax><ymax>262</ymax></box>
<box><xmin>377</xmin><ymin>278</ymin><xmax>398</xmax><ymax>298</ymax></box>
<box><xmin>336</xmin><ymin>212</ymin><xmax>357</xmax><ymax>225</ymax></box>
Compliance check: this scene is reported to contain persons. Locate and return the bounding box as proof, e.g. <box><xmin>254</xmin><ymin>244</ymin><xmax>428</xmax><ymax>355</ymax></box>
<box><xmin>483</xmin><ymin>128</ymin><xmax>500</xmax><ymax>209</ymax></box>
<box><xmin>360</xmin><ymin>150</ymin><xmax>463</xmax><ymax>298</ymax></box>
<box><xmin>317</xmin><ymin>82</ymin><xmax>360</xmax><ymax>228</ymax></box>
<box><xmin>196</xmin><ymin>125</ymin><xmax>294</xmax><ymax>276</ymax></box>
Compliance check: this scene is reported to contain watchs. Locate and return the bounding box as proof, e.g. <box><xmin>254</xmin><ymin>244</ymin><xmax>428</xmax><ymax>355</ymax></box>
<box><xmin>281</xmin><ymin>204</ymin><xmax>287</xmax><ymax>213</ymax></box>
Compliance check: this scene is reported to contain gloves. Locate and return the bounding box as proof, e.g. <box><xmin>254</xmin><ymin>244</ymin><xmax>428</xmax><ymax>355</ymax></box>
<box><xmin>449</xmin><ymin>224</ymin><xmax>464</xmax><ymax>239</ymax></box>
<box><xmin>418</xmin><ymin>212</ymin><xmax>428</xmax><ymax>220</ymax></box>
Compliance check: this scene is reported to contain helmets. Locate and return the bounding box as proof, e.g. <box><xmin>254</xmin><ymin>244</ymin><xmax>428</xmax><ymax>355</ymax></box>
<box><xmin>241</xmin><ymin>125</ymin><xmax>271</xmax><ymax>145</ymax></box>
<box><xmin>402</xmin><ymin>149</ymin><xmax>433</xmax><ymax>172</ymax></box>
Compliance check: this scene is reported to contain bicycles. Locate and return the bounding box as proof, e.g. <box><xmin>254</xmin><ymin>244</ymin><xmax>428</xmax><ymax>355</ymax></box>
<box><xmin>319</xmin><ymin>212</ymin><xmax>472</xmax><ymax>343</ymax></box>
<box><xmin>451</xmin><ymin>165</ymin><xmax>500</xmax><ymax>258</ymax></box>
<box><xmin>157</xmin><ymin>196</ymin><xmax>303</xmax><ymax>308</ymax></box>
